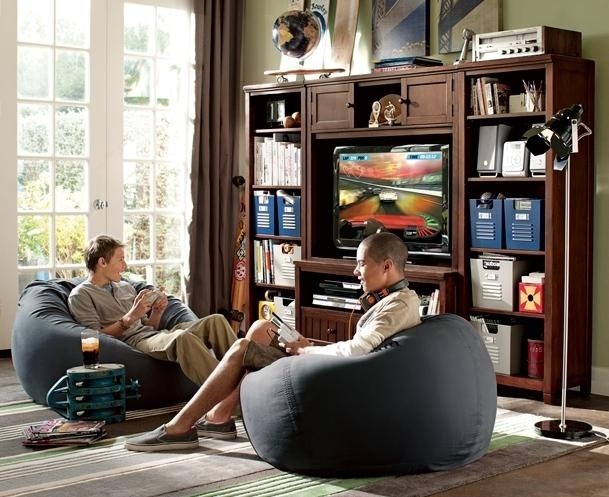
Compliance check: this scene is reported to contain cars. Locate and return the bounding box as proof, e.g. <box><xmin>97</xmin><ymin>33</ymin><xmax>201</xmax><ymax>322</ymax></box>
<box><xmin>339</xmin><ymin>187</ymin><xmax>382</xmax><ymax>208</ymax></box>
<box><xmin>378</xmin><ymin>188</ymin><xmax>398</xmax><ymax>201</ymax></box>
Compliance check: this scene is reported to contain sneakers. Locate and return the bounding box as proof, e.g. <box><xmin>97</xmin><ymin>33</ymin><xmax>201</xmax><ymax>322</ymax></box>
<box><xmin>124</xmin><ymin>423</ymin><xmax>199</xmax><ymax>452</ymax></box>
<box><xmin>194</xmin><ymin>412</ymin><xmax>238</xmax><ymax>441</ymax></box>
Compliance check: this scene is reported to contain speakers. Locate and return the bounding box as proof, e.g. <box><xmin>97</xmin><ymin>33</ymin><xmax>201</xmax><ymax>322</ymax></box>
<box><xmin>476</xmin><ymin>123</ymin><xmax>511</xmax><ymax>178</ymax></box>
<box><xmin>529</xmin><ymin>145</ymin><xmax>546</xmax><ymax>178</ymax></box>
<box><xmin>501</xmin><ymin>140</ymin><xmax>527</xmax><ymax>177</ymax></box>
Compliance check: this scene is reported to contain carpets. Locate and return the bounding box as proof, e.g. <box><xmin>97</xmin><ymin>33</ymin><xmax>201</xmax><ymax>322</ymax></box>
<box><xmin>0</xmin><ymin>399</ymin><xmax>609</xmax><ymax>496</ymax></box>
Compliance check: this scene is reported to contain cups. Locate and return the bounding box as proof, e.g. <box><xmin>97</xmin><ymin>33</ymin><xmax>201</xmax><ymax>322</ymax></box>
<box><xmin>80</xmin><ymin>329</ymin><xmax>100</xmax><ymax>369</ymax></box>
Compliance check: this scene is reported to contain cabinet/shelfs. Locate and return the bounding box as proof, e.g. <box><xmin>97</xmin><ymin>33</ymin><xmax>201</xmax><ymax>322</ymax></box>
<box><xmin>456</xmin><ymin>55</ymin><xmax>594</xmax><ymax>405</ymax></box>
<box><xmin>291</xmin><ymin>255</ymin><xmax>456</xmax><ymax>345</ymax></box>
<box><xmin>305</xmin><ymin>74</ymin><xmax>456</xmax><ymax>144</ymax></box>
<box><xmin>243</xmin><ymin>85</ymin><xmax>305</xmax><ymax>337</ymax></box>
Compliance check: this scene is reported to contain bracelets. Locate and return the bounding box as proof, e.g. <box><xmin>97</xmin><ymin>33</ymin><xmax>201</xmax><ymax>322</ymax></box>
<box><xmin>119</xmin><ymin>318</ymin><xmax>129</xmax><ymax>331</ymax></box>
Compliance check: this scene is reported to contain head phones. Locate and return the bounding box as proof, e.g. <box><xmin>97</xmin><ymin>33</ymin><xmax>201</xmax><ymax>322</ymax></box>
<box><xmin>359</xmin><ymin>278</ymin><xmax>409</xmax><ymax>312</ymax></box>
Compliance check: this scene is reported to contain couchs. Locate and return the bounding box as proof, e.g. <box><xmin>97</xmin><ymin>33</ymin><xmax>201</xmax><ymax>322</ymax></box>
<box><xmin>11</xmin><ymin>276</ymin><xmax>202</xmax><ymax>414</ymax></box>
<box><xmin>240</xmin><ymin>312</ymin><xmax>497</xmax><ymax>472</ymax></box>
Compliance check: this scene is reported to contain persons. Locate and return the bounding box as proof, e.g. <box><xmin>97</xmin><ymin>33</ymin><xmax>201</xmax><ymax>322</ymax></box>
<box><xmin>123</xmin><ymin>232</ymin><xmax>421</xmax><ymax>452</ymax></box>
<box><xmin>385</xmin><ymin>101</ymin><xmax>396</xmax><ymax>119</ymax></box>
<box><xmin>68</xmin><ymin>235</ymin><xmax>244</xmax><ymax>416</ymax></box>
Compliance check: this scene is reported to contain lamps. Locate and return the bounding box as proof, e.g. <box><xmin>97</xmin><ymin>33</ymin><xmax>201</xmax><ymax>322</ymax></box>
<box><xmin>525</xmin><ymin>102</ymin><xmax>594</xmax><ymax>441</ymax></box>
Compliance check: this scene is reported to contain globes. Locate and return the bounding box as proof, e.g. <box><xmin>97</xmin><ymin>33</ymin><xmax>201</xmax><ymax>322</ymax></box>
<box><xmin>263</xmin><ymin>11</ymin><xmax>345</xmax><ymax>83</ymax></box>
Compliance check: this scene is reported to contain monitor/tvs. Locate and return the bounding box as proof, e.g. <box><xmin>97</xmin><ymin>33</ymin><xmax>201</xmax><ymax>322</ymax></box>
<box><xmin>332</xmin><ymin>143</ymin><xmax>450</xmax><ymax>264</ymax></box>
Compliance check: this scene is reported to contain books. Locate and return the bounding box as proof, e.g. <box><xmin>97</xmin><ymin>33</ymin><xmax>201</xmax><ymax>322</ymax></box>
<box><xmin>20</xmin><ymin>419</ymin><xmax>109</xmax><ymax>446</ymax></box>
<box><xmin>371</xmin><ymin>55</ymin><xmax>442</xmax><ymax>74</ymax></box>
<box><xmin>426</xmin><ymin>288</ymin><xmax>440</xmax><ymax>316</ymax></box>
<box><xmin>253</xmin><ymin>133</ymin><xmax>302</xmax><ymax>187</ymax></box>
<box><xmin>252</xmin><ymin>238</ymin><xmax>280</xmax><ymax>284</ymax></box>
<box><xmin>469</xmin><ymin>77</ymin><xmax>511</xmax><ymax>115</ymax></box>
